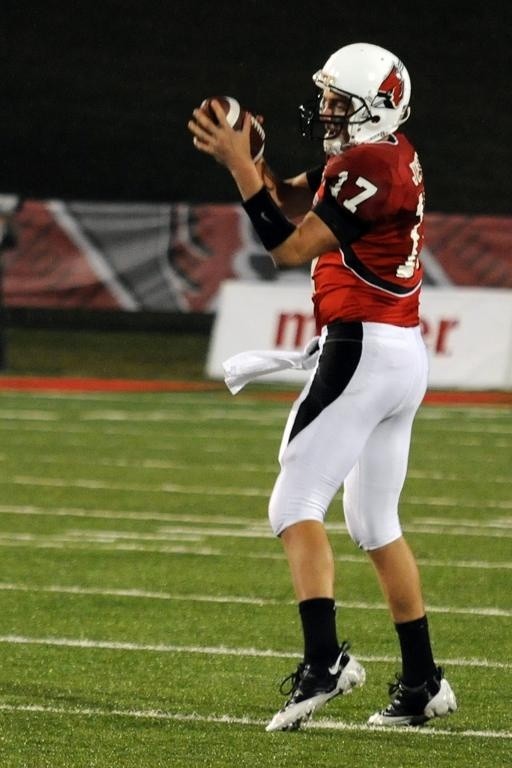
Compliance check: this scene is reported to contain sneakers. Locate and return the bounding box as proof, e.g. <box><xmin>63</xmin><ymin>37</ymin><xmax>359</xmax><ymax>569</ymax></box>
<box><xmin>264</xmin><ymin>649</ymin><xmax>366</xmax><ymax>733</ymax></box>
<box><xmin>368</xmin><ymin>668</ymin><xmax>457</xmax><ymax>727</ymax></box>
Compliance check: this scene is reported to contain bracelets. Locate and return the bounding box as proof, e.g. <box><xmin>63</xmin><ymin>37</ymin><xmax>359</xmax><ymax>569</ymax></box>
<box><xmin>239</xmin><ymin>186</ymin><xmax>298</xmax><ymax>256</ymax></box>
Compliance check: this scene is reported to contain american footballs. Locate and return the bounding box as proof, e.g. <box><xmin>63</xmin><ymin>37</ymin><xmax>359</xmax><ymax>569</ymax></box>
<box><xmin>199</xmin><ymin>96</ymin><xmax>265</xmax><ymax>164</ymax></box>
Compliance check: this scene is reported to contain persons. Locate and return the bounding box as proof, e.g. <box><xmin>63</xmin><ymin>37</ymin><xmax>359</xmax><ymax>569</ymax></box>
<box><xmin>189</xmin><ymin>42</ymin><xmax>458</xmax><ymax>732</ymax></box>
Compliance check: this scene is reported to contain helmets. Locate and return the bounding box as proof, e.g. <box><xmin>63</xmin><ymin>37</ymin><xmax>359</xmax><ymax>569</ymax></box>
<box><xmin>299</xmin><ymin>43</ymin><xmax>412</xmax><ymax>156</ymax></box>
